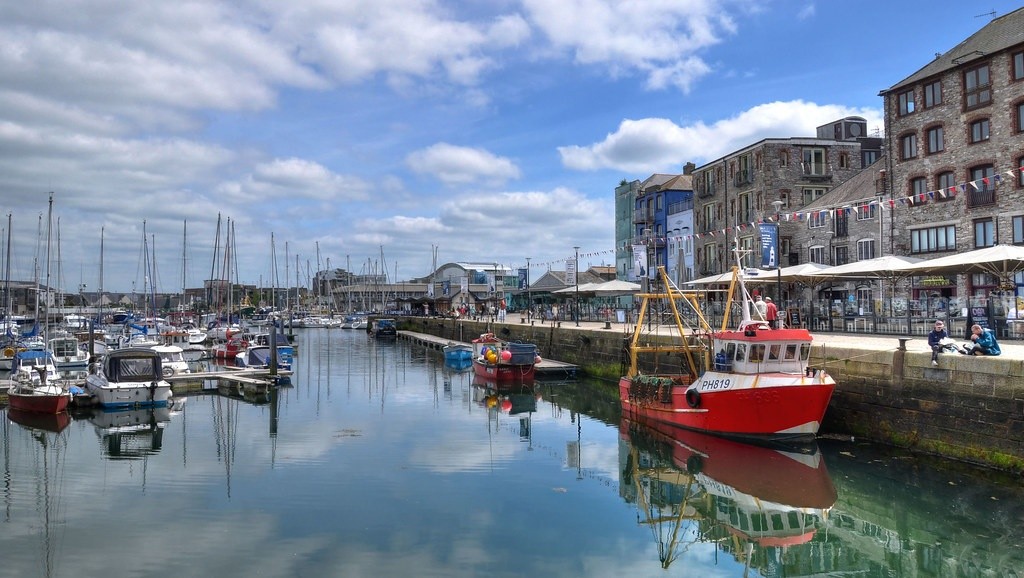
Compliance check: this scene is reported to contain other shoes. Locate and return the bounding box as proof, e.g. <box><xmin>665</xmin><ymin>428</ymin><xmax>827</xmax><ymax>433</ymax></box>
<box><xmin>975</xmin><ymin>351</ymin><xmax>983</xmax><ymax>356</ymax></box>
<box><xmin>931</xmin><ymin>360</ymin><xmax>938</xmax><ymax>366</ymax></box>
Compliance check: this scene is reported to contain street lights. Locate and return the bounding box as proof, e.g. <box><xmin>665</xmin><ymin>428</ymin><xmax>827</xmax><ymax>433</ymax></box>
<box><xmin>492</xmin><ymin>262</ymin><xmax>500</xmax><ymax>320</ymax></box>
<box><xmin>643</xmin><ymin>228</ymin><xmax>652</xmax><ymax>331</ymax></box>
<box><xmin>524</xmin><ymin>257</ymin><xmax>533</xmax><ymax>323</ymax></box>
<box><xmin>572</xmin><ymin>246</ymin><xmax>581</xmax><ymax>326</ymax></box>
<box><xmin>771</xmin><ymin>200</ymin><xmax>785</xmax><ymax>310</ymax></box>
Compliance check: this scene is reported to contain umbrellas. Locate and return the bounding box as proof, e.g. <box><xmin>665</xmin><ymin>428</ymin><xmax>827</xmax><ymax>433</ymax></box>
<box><xmin>681</xmin><ymin>242</ymin><xmax>1024</xmax><ymax>318</ymax></box>
<box><xmin>550</xmin><ymin>278</ymin><xmax>658</xmax><ymax>294</ymax></box>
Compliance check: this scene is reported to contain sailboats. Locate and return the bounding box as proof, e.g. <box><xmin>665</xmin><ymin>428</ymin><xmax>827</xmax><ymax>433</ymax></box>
<box><xmin>1</xmin><ymin>188</ymin><xmax>371</xmax><ymax>429</ymax></box>
<box><xmin>92</xmin><ymin>426</ymin><xmax>166</xmax><ymax>495</ymax></box>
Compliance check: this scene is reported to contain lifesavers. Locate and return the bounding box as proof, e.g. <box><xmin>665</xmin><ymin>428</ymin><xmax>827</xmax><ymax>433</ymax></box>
<box><xmin>685</xmin><ymin>389</ymin><xmax>700</xmax><ymax>408</ymax></box>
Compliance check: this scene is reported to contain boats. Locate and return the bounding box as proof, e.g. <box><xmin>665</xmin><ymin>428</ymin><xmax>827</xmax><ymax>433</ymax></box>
<box><xmin>472</xmin><ymin>332</ymin><xmax>536</xmax><ymax>379</ymax></box>
<box><xmin>443</xmin><ymin>340</ymin><xmax>472</xmax><ymax>360</ymax></box>
<box><xmin>443</xmin><ymin>358</ymin><xmax>474</xmax><ymax>375</ymax></box>
<box><xmin>618</xmin><ymin>264</ymin><xmax>836</xmax><ymax>444</ymax></box>
<box><xmin>471</xmin><ymin>374</ymin><xmax>537</xmax><ymax>416</ymax></box>
<box><xmin>619</xmin><ymin>408</ymin><xmax>839</xmax><ymax>578</ymax></box>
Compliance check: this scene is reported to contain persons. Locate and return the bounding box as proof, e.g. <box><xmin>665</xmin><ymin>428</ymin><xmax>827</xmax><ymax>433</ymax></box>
<box><xmin>752</xmin><ymin>295</ymin><xmax>768</xmax><ymax>321</ymax></box>
<box><xmin>764</xmin><ymin>297</ymin><xmax>777</xmax><ymax>330</ymax></box>
<box><xmin>963</xmin><ymin>324</ymin><xmax>1001</xmax><ymax>355</ymax></box>
<box><xmin>928</xmin><ymin>321</ymin><xmax>966</xmax><ymax>365</ymax></box>
<box><xmin>402</xmin><ymin>297</ymin><xmax>719</xmax><ymax>324</ymax></box>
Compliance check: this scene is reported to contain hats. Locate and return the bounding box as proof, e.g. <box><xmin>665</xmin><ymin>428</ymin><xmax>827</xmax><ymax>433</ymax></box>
<box><xmin>756</xmin><ymin>295</ymin><xmax>762</xmax><ymax>299</ymax></box>
<box><xmin>765</xmin><ymin>297</ymin><xmax>772</xmax><ymax>300</ymax></box>
<box><xmin>935</xmin><ymin>321</ymin><xmax>944</xmax><ymax>327</ymax></box>
